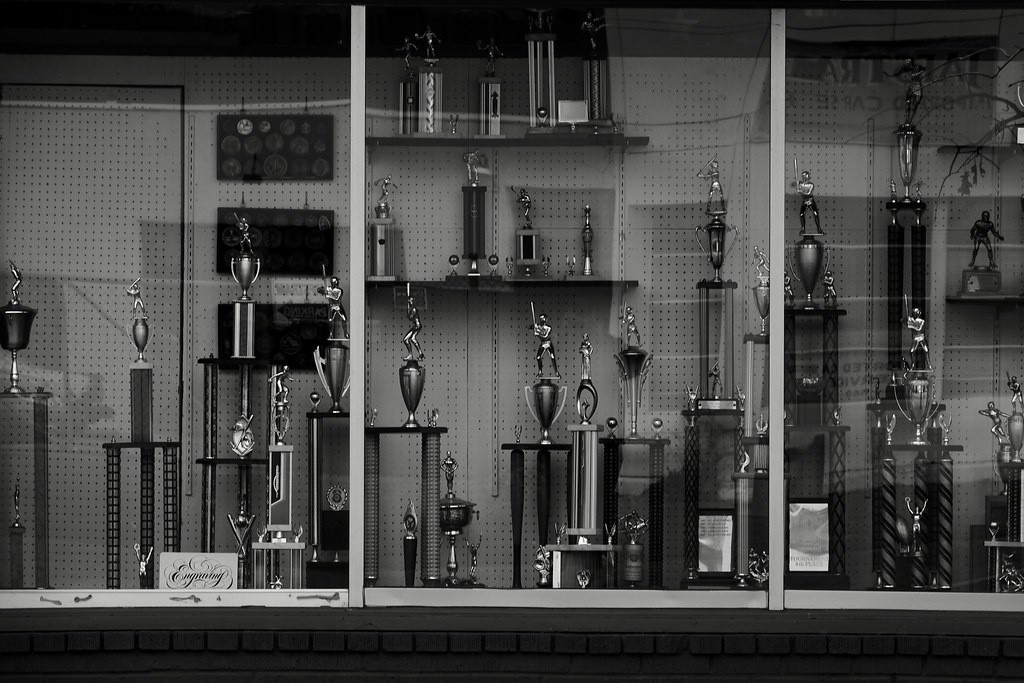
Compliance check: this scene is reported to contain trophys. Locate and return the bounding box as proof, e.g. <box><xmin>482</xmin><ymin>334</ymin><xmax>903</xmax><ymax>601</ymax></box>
<box><xmin>0</xmin><ymin>187</ymin><xmax>351</xmax><ymax>589</ymax></box>
<box><xmin>784</xmin><ymin>55</ymin><xmax>1024</xmax><ymax>592</ymax></box>
<box><xmin>363</xmin><ymin>12</ymin><xmax>770</xmax><ymax>588</ymax></box>
